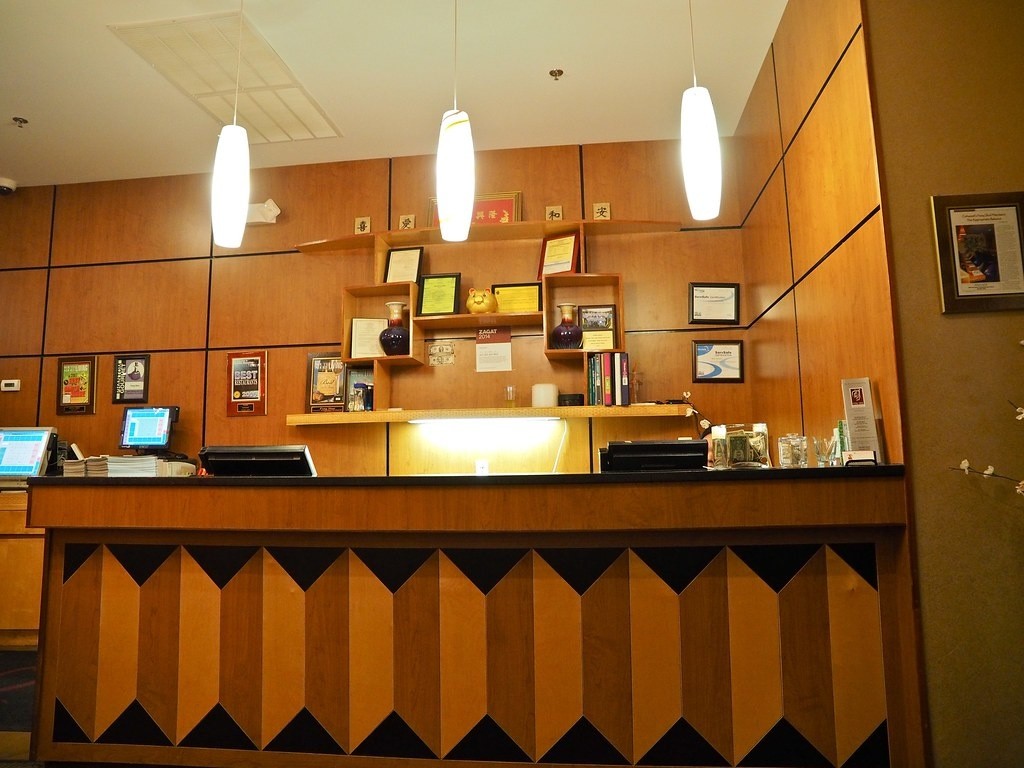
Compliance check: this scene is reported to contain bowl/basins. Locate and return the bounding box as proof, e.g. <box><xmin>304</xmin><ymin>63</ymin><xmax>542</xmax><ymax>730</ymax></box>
<box><xmin>531</xmin><ymin>384</ymin><xmax>584</xmax><ymax>407</ymax></box>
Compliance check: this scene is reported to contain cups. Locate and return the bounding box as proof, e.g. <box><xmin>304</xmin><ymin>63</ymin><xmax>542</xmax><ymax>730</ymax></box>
<box><xmin>814</xmin><ymin>441</ymin><xmax>837</xmax><ymax>467</ymax></box>
<box><xmin>503</xmin><ymin>384</ymin><xmax>516</xmax><ymax>408</ymax></box>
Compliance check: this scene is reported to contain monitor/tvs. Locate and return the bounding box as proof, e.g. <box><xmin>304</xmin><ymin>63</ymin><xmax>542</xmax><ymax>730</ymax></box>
<box><xmin>598</xmin><ymin>441</ymin><xmax>708</xmax><ymax>473</ymax></box>
<box><xmin>117</xmin><ymin>406</ymin><xmax>179</xmax><ymax>455</ymax></box>
<box><xmin>199</xmin><ymin>445</ymin><xmax>317</xmax><ymax>478</ymax></box>
<box><xmin>0</xmin><ymin>427</ymin><xmax>58</xmax><ymax>491</ymax></box>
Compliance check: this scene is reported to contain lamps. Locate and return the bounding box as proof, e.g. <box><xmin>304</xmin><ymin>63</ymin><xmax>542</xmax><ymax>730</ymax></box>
<box><xmin>436</xmin><ymin>0</ymin><xmax>475</xmax><ymax>242</ymax></box>
<box><xmin>680</xmin><ymin>0</ymin><xmax>723</xmax><ymax>222</ymax></box>
<box><xmin>210</xmin><ymin>0</ymin><xmax>251</xmax><ymax>249</ymax></box>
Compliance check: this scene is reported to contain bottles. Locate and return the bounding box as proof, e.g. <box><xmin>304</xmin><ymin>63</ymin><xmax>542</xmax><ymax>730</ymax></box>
<box><xmin>550</xmin><ymin>303</ymin><xmax>583</xmax><ymax>349</ymax></box>
<box><xmin>778</xmin><ymin>433</ymin><xmax>808</xmax><ymax>469</ymax></box>
<box><xmin>352</xmin><ymin>382</ymin><xmax>374</xmax><ymax>412</ymax></box>
<box><xmin>379</xmin><ymin>302</ymin><xmax>409</xmax><ymax>356</ymax></box>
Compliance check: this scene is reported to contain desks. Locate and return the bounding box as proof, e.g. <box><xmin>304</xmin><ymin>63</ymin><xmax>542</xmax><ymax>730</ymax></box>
<box><xmin>23</xmin><ymin>461</ymin><xmax>938</xmax><ymax>768</ymax></box>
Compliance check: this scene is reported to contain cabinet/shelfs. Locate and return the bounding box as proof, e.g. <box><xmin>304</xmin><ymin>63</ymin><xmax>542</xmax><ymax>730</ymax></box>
<box><xmin>284</xmin><ymin>216</ymin><xmax>694</xmax><ymax>425</ymax></box>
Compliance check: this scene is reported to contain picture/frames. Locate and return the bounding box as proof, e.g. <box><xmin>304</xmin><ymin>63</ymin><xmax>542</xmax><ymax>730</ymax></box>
<box><xmin>692</xmin><ymin>340</ymin><xmax>744</xmax><ymax>383</ymax></box>
<box><xmin>304</xmin><ymin>352</ymin><xmax>348</xmax><ymax>414</ymax></box>
<box><xmin>926</xmin><ymin>191</ymin><xmax>1024</xmax><ymax>316</ymax></box>
<box><xmin>56</xmin><ymin>356</ymin><xmax>97</xmax><ymax>414</ymax></box>
<box><xmin>688</xmin><ymin>281</ymin><xmax>740</xmax><ymax>325</ymax></box>
<box><xmin>578</xmin><ymin>304</ymin><xmax>616</xmax><ymax>350</ymax></box>
<box><xmin>537</xmin><ymin>231</ymin><xmax>581</xmax><ymax>281</ymax></box>
<box><xmin>111</xmin><ymin>354</ymin><xmax>150</xmax><ymax>404</ymax></box>
<box><xmin>383</xmin><ymin>247</ymin><xmax>424</xmax><ymax>283</ymax></box>
<box><xmin>416</xmin><ymin>272</ymin><xmax>461</xmax><ymax>316</ymax></box>
<box><xmin>490</xmin><ymin>282</ymin><xmax>543</xmax><ymax>312</ymax></box>
